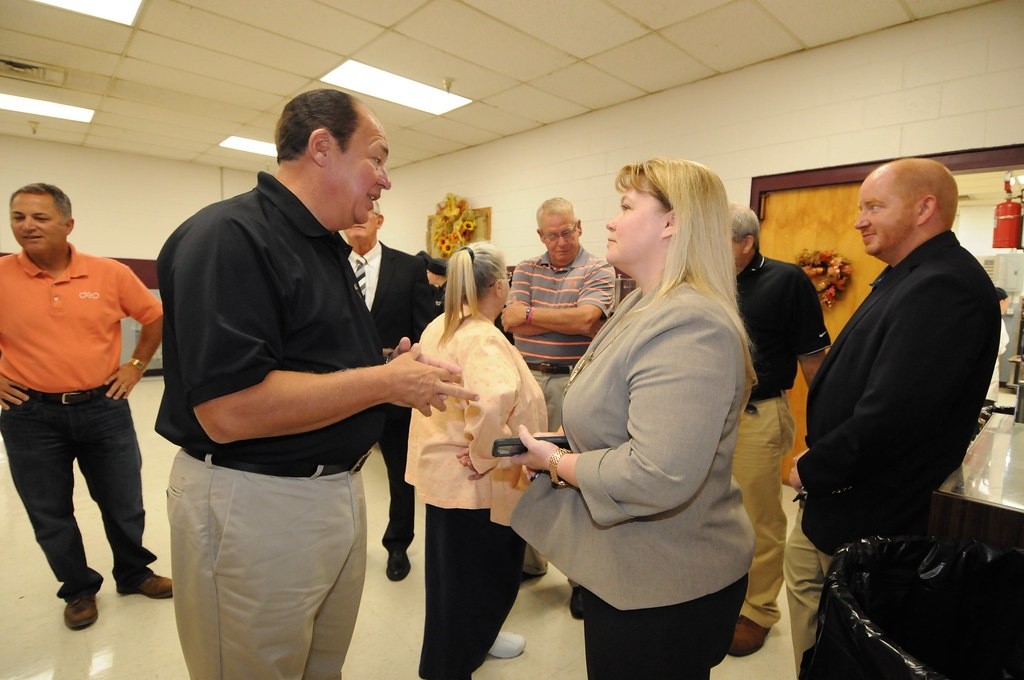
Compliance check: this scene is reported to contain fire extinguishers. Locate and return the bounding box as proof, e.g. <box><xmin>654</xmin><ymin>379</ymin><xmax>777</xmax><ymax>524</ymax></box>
<box><xmin>992</xmin><ymin>171</ymin><xmax>1024</xmax><ymax>248</ymax></box>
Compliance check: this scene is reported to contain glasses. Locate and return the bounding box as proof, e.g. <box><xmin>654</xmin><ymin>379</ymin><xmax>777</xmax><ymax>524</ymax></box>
<box><xmin>542</xmin><ymin>221</ymin><xmax>577</xmax><ymax>240</ymax></box>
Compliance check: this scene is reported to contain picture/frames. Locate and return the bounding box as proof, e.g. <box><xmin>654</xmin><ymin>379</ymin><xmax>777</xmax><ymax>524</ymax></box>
<box><xmin>427</xmin><ymin>207</ymin><xmax>491</xmax><ymax>258</ymax></box>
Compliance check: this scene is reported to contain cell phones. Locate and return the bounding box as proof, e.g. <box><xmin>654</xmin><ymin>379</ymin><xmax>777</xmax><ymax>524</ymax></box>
<box><xmin>491</xmin><ymin>436</ymin><xmax>571</xmax><ymax>458</ymax></box>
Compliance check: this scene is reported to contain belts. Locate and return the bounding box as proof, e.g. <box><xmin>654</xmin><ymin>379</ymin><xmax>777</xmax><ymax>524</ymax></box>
<box><xmin>29</xmin><ymin>385</ymin><xmax>109</xmax><ymax>405</ymax></box>
<box><xmin>745</xmin><ymin>390</ymin><xmax>787</xmax><ymax>413</ymax></box>
<box><xmin>185</xmin><ymin>450</ymin><xmax>371</xmax><ymax>478</ymax></box>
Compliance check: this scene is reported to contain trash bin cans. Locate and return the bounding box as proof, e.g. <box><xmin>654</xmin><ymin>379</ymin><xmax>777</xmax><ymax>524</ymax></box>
<box><xmin>831</xmin><ymin>535</ymin><xmax>1024</xmax><ymax>680</ymax></box>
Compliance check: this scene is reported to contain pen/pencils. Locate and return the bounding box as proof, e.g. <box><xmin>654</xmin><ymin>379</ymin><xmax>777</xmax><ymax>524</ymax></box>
<box><xmin>530</xmin><ymin>470</ymin><xmax>541</xmax><ymax>481</ymax></box>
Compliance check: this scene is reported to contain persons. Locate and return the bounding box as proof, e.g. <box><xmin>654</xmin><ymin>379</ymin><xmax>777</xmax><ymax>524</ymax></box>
<box><xmin>0</xmin><ymin>183</ymin><xmax>172</xmax><ymax>629</ymax></box>
<box><xmin>349</xmin><ymin>200</ymin><xmax>433</xmax><ymax>581</ymax></box>
<box><xmin>983</xmin><ymin>287</ymin><xmax>1009</xmax><ymax>408</ymax></box>
<box><xmin>155</xmin><ymin>90</ymin><xmax>479</xmax><ymax>680</ymax></box>
<box><xmin>728</xmin><ymin>205</ymin><xmax>832</xmax><ymax>656</ymax></box>
<box><xmin>427</xmin><ymin>257</ymin><xmax>447</xmax><ymax>318</ymax></box>
<box><xmin>405</xmin><ymin>243</ymin><xmax>548</xmax><ymax>680</ymax></box>
<box><xmin>511</xmin><ymin>160</ymin><xmax>755</xmax><ymax>679</ymax></box>
<box><xmin>500</xmin><ymin>198</ymin><xmax>616</xmax><ymax>615</ymax></box>
<box><xmin>785</xmin><ymin>156</ymin><xmax>1000</xmax><ymax>680</ymax></box>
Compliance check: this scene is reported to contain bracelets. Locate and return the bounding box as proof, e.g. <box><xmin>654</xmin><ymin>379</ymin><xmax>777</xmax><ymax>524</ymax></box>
<box><xmin>528</xmin><ymin>307</ymin><xmax>535</xmax><ymax>325</ymax></box>
<box><xmin>385</xmin><ymin>357</ymin><xmax>389</xmax><ymax>364</ymax></box>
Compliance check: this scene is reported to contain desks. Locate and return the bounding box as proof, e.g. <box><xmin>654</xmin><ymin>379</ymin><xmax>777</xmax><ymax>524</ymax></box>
<box><xmin>929</xmin><ymin>412</ymin><xmax>1024</xmax><ymax>548</ymax></box>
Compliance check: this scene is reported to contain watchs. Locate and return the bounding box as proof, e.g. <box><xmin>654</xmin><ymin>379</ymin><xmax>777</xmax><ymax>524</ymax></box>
<box><xmin>550</xmin><ymin>449</ymin><xmax>572</xmax><ymax>486</ymax></box>
<box><xmin>526</xmin><ymin>308</ymin><xmax>531</xmax><ymax>320</ymax></box>
<box><xmin>130</xmin><ymin>358</ymin><xmax>144</xmax><ymax>369</ymax></box>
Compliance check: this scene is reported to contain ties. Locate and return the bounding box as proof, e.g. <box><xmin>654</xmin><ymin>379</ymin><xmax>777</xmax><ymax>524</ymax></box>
<box><xmin>354</xmin><ymin>258</ymin><xmax>367</xmax><ymax>302</ymax></box>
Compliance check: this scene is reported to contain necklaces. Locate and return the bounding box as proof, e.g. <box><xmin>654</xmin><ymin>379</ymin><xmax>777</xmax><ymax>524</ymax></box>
<box><xmin>570</xmin><ymin>351</ymin><xmax>593</xmax><ymax>382</ymax></box>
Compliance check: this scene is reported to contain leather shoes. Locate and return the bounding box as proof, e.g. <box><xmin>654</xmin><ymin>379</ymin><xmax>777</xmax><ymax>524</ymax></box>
<box><xmin>386</xmin><ymin>549</ymin><xmax>411</xmax><ymax>581</ymax></box>
<box><xmin>728</xmin><ymin>615</ymin><xmax>770</xmax><ymax>659</ymax></box>
<box><xmin>114</xmin><ymin>574</ymin><xmax>173</xmax><ymax>598</ymax></box>
<box><xmin>63</xmin><ymin>599</ymin><xmax>100</xmax><ymax>629</ymax></box>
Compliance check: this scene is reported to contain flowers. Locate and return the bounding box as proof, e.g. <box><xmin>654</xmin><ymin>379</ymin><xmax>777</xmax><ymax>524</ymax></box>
<box><xmin>798</xmin><ymin>249</ymin><xmax>853</xmax><ymax>308</ymax></box>
<box><xmin>433</xmin><ymin>192</ymin><xmax>476</xmax><ymax>259</ymax></box>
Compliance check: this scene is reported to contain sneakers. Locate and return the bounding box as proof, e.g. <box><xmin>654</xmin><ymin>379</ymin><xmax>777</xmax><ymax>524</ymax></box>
<box><xmin>489</xmin><ymin>630</ymin><xmax>525</xmax><ymax>658</ymax></box>
